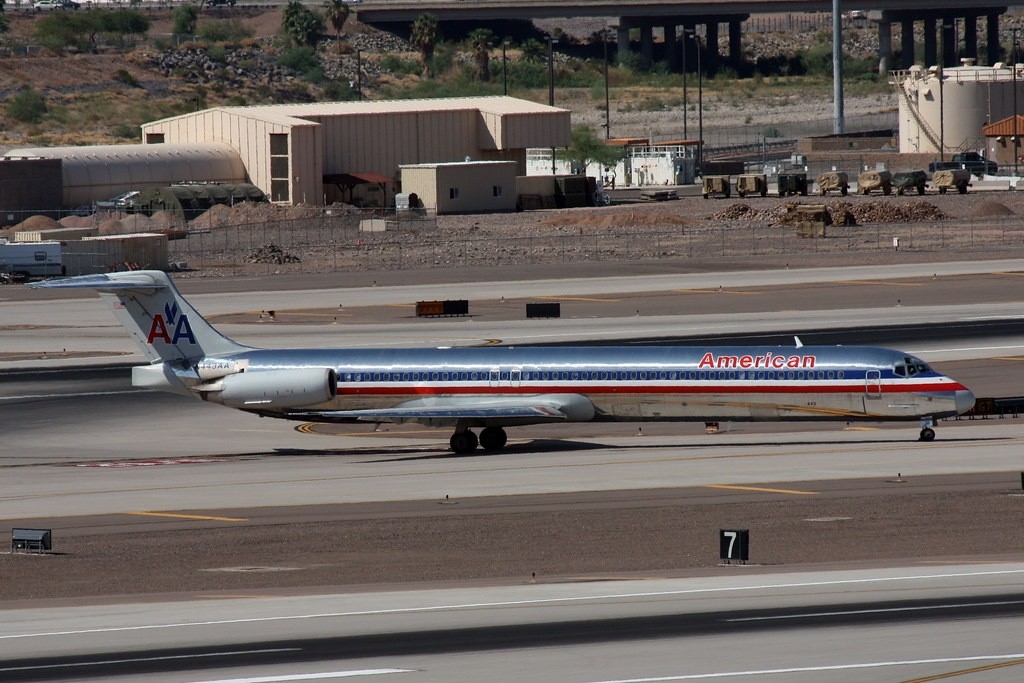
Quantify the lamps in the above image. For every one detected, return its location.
[894,237,899,247]
[11,527,52,555]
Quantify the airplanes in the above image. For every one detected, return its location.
[23,270,978,451]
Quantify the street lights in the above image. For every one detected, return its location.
[1009,27,1021,172]
[549,40,560,106]
[603,32,618,139]
[691,34,703,168]
[502,40,510,95]
[683,28,695,140]
[358,49,365,100]
[940,24,954,161]
[543,35,554,106]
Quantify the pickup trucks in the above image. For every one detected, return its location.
[930,151,997,178]
